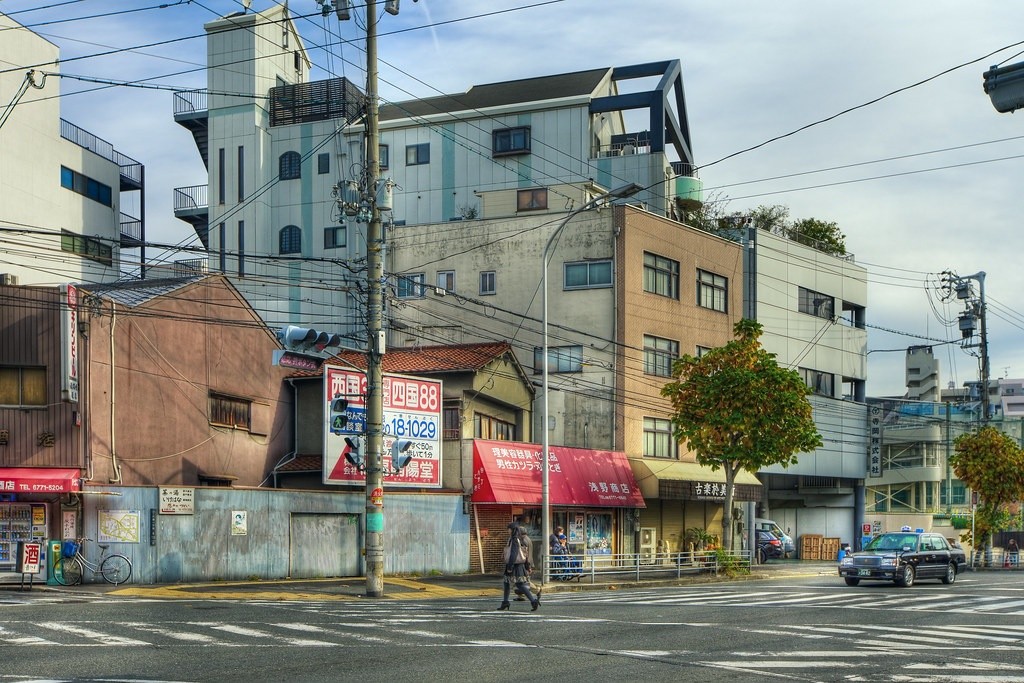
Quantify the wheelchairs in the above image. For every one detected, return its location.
[551,556,583,581]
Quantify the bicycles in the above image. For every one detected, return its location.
[53,536,132,587]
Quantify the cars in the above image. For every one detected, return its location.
[839,525,967,587]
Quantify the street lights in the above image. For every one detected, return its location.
[541,182,646,586]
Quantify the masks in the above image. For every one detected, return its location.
[558,533,563,536]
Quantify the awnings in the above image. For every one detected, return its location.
[471,439,647,508]
[626,456,763,502]
[0,468,80,492]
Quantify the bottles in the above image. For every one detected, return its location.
[0,508,31,561]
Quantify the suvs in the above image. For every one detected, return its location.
[743,529,785,564]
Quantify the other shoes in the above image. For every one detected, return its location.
[537,587,542,599]
[513,596,526,601]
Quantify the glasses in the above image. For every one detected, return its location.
[563,539,565,540]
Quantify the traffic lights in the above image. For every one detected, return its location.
[345,433,359,466]
[392,438,412,468]
[281,324,344,358]
[331,396,348,430]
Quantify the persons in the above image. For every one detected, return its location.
[836,546,850,562]
[496,523,542,612]
[784,543,791,558]
[592,516,599,531]
[1007,539,1019,565]
[549,526,582,575]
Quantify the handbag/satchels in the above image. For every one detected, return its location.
[501,538,526,564]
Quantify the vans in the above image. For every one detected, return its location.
[737,517,794,554]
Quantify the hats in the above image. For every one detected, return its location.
[560,535,567,539]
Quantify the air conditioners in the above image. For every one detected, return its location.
[0,274,19,285]
[634,527,656,565]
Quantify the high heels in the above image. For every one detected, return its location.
[497,601,510,610]
[531,599,541,611]
